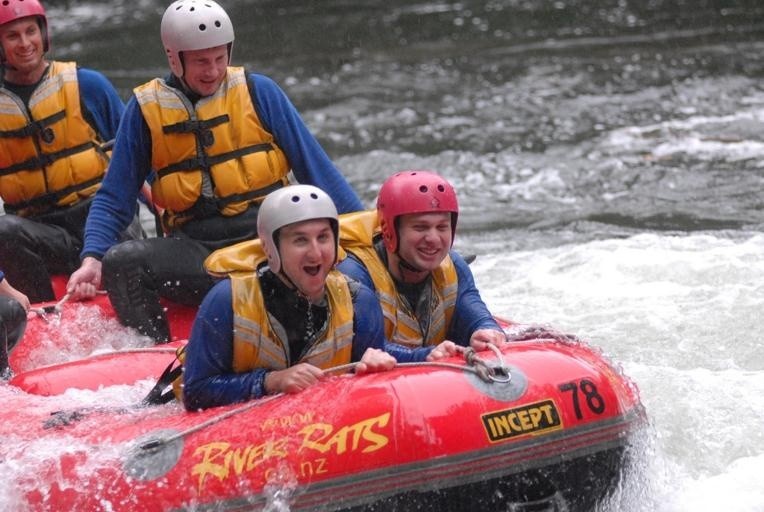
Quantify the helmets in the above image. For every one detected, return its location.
[377,169,458,254]
[256,183,339,275]
[161,0,234,78]
[0,0,50,52]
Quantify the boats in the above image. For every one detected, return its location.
[0,272,656,511]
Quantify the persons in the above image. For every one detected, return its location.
[165,183,398,414]
[1,0,155,307]
[335,169,507,364]
[64,1,369,347]
[0,271,31,375]
